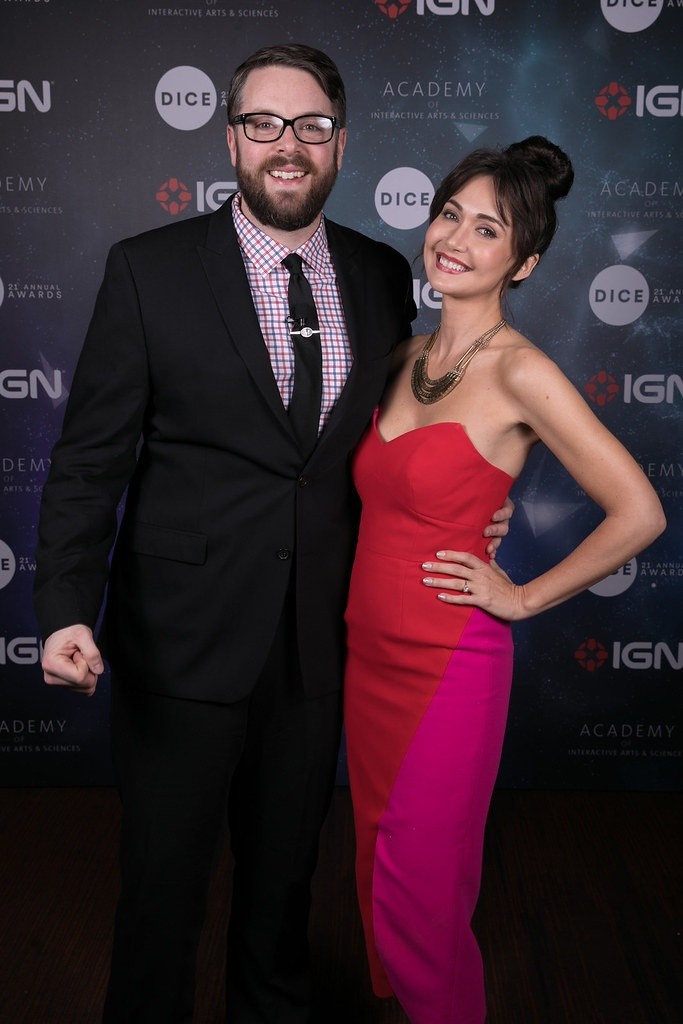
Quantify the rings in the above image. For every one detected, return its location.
[463,580,469,592]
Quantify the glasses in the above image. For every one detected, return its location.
[230,113,340,145]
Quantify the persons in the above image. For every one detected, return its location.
[32,43,419,1024]
[342,136,668,1024]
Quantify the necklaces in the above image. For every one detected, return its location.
[411,320,508,404]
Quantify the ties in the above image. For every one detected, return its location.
[281,254,322,454]
